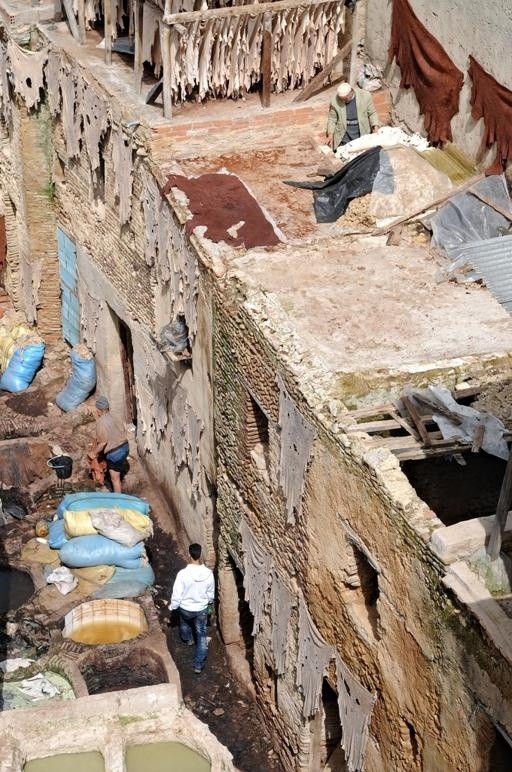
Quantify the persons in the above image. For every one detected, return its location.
[87,395,129,493]
[325,82,378,151]
[167,543,215,673]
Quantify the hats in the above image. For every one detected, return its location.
[337,83,351,96]
[95,396,109,409]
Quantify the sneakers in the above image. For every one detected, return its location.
[194,667,201,673]
[187,640,194,646]
